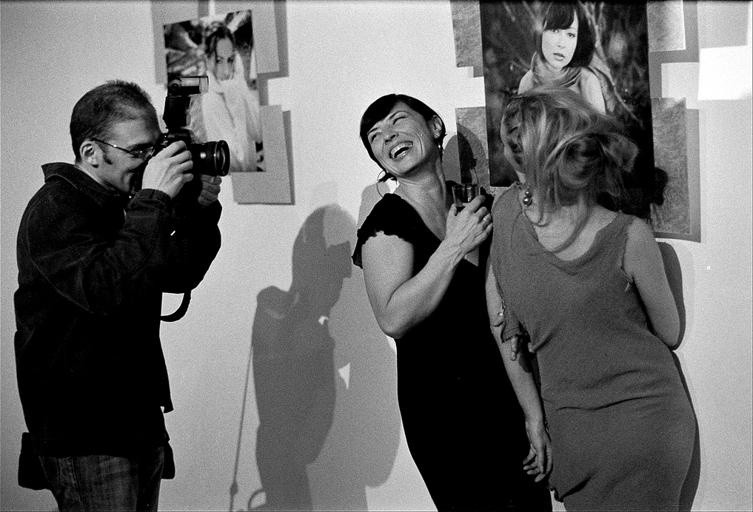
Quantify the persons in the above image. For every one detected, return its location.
[485,87,696,512]
[168,22,262,171]
[351,94,553,512]
[13,82,221,512]
[517,1,606,116]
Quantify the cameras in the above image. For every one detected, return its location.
[161,69,231,195]
[443,178,494,219]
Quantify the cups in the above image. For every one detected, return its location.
[450,185,482,214]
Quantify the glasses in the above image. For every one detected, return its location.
[88,137,155,161]
